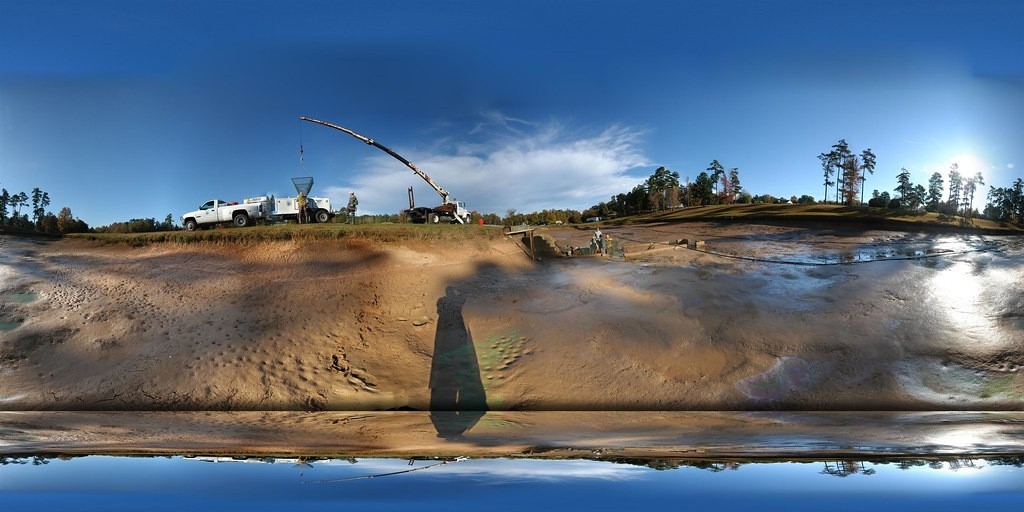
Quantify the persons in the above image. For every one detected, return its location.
[296,192,309,224]
[345,191,358,225]
[590,227,613,255]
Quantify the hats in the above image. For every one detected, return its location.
[299,192,304,196]
[349,191,354,195]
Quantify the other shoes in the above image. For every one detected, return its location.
[344,222,350,224]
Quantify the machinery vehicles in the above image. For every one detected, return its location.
[299,115,471,224]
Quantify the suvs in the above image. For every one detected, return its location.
[535,220,548,226]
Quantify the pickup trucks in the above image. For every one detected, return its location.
[180,199,263,232]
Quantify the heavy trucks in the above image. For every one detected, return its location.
[242,193,332,225]
